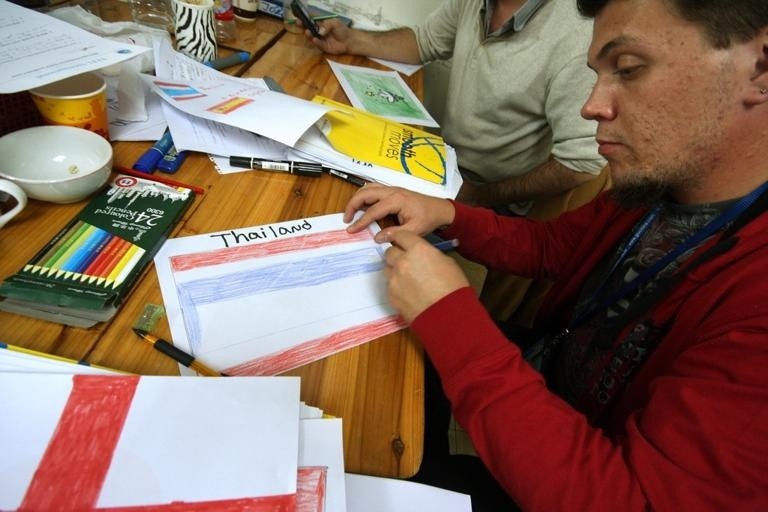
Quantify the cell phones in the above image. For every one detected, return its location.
[290,0,321,40]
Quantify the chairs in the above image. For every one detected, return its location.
[474,161,618,328]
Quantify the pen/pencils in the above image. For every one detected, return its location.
[284,14,339,24]
[22,217,149,291]
[323,168,368,187]
[133,327,226,376]
[433,239,460,251]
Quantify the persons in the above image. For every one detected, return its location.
[297,0,608,213]
[342,0,767,512]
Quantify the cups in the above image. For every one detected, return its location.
[26,72,110,142]
[232,1,258,23]
[174,1,217,63]
[128,1,174,35]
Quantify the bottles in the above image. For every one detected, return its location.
[214,0,239,44]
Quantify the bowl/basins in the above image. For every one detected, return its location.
[0,125,112,205]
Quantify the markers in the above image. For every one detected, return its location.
[132,131,174,174]
[230,157,321,176]
[203,52,250,70]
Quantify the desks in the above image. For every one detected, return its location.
[2,0,427,483]
